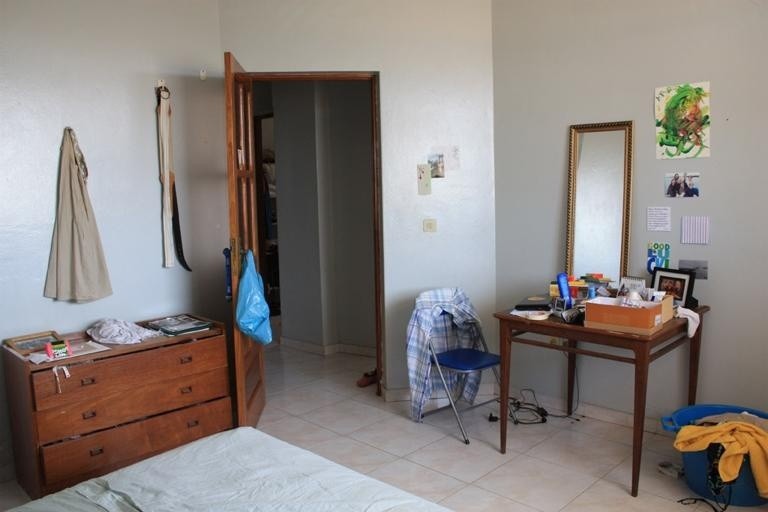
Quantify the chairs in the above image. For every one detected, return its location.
[415,287,518,446]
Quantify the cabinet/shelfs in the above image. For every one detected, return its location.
[2,313,236,499]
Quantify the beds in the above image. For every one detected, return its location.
[0,426,454,512]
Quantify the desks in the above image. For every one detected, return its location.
[492,304,711,497]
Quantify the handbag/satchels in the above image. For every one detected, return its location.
[235,248,272,346]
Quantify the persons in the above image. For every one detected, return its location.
[667,173,699,197]
[660,277,683,300]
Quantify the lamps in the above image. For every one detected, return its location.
[428,151,446,180]
[650,266,696,308]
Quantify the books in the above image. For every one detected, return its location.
[144,314,212,338]
[515,294,552,311]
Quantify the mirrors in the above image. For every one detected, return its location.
[566,120,633,278]
